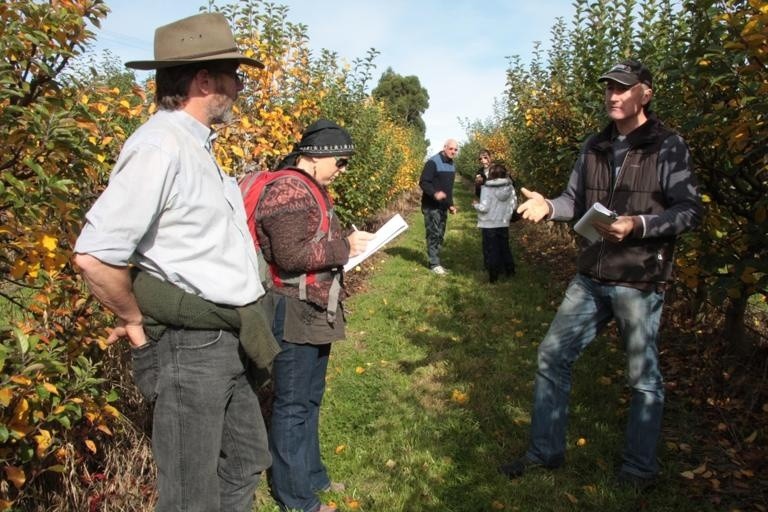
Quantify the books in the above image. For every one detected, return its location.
[341,212,409,274]
[573,201,615,244]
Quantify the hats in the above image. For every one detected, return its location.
[125,12,265,70]
[596,59,654,87]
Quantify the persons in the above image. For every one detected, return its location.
[475,149,492,205]
[255,121,351,510]
[69,15,270,510]
[468,161,519,284]
[503,60,702,490]
[416,136,461,278]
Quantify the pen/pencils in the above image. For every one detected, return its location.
[351,223,358,232]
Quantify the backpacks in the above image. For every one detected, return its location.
[236,169,330,286]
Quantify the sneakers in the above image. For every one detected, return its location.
[499,454,534,479]
[613,472,655,494]
[329,482,348,493]
[433,266,444,274]
[318,505,339,512]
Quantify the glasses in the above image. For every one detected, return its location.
[335,156,348,168]
